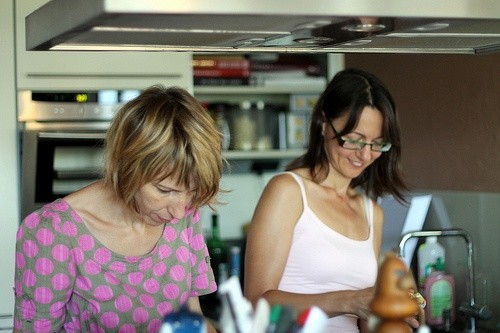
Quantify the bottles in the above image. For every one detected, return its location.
[206,212,227,281]
[418,236,446,282]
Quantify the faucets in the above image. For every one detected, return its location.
[397,228,480,333]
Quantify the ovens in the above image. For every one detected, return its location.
[14,50,195,224]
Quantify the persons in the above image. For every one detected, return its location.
[13,84,234,333]
[243,68,419,333]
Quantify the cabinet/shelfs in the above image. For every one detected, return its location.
[193,54,346,159]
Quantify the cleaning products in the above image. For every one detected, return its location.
[422,255,457,327]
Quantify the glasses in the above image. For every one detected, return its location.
[328,119,393,152]
